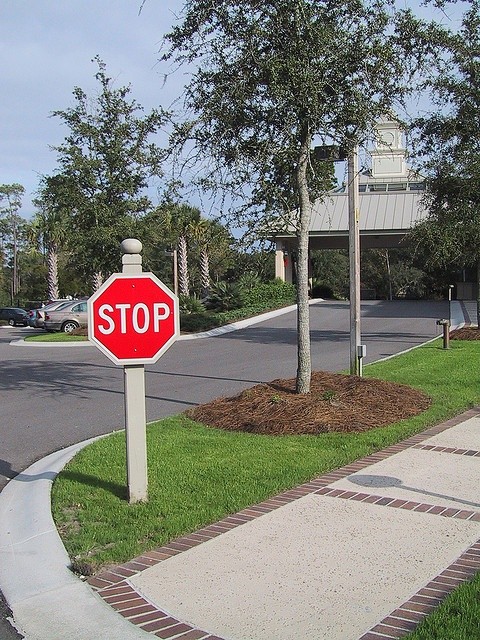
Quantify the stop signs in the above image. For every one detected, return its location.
[87,272,180,366]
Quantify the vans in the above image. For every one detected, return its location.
[0,307,27,326]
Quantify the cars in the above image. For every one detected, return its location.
[29,310,38,328]
[43,300,87,333]
[34,301,74,332]
[23,312,30,326]
